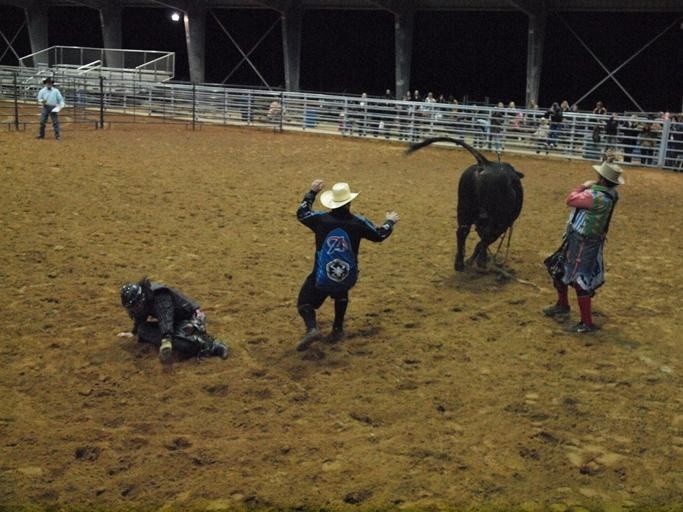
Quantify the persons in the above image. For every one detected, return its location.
[339,91,524,150]
[526,100,683,169]
[118,280,229,361]
[295,179,399,351]
[269,95,286,121]
[306,105,319,128]
[545,161,624,334]
[36,78,64,140]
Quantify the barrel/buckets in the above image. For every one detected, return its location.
[305,110,317,127]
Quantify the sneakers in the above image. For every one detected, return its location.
[214,339,229,361]
[543,304,572,316]
[320,328,345,345]
[564,322,597,333]
[295,328,319,352]
[34,134,62,142]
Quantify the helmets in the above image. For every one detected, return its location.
[120,283,148,315]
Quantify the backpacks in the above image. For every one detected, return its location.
[313,213,363,293]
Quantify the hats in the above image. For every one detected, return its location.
[42,77,55,85]
[319,181,362,210]
[592,159,626,184]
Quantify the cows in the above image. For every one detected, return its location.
[405,137,524,272]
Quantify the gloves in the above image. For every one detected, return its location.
[158,338,173,363]
